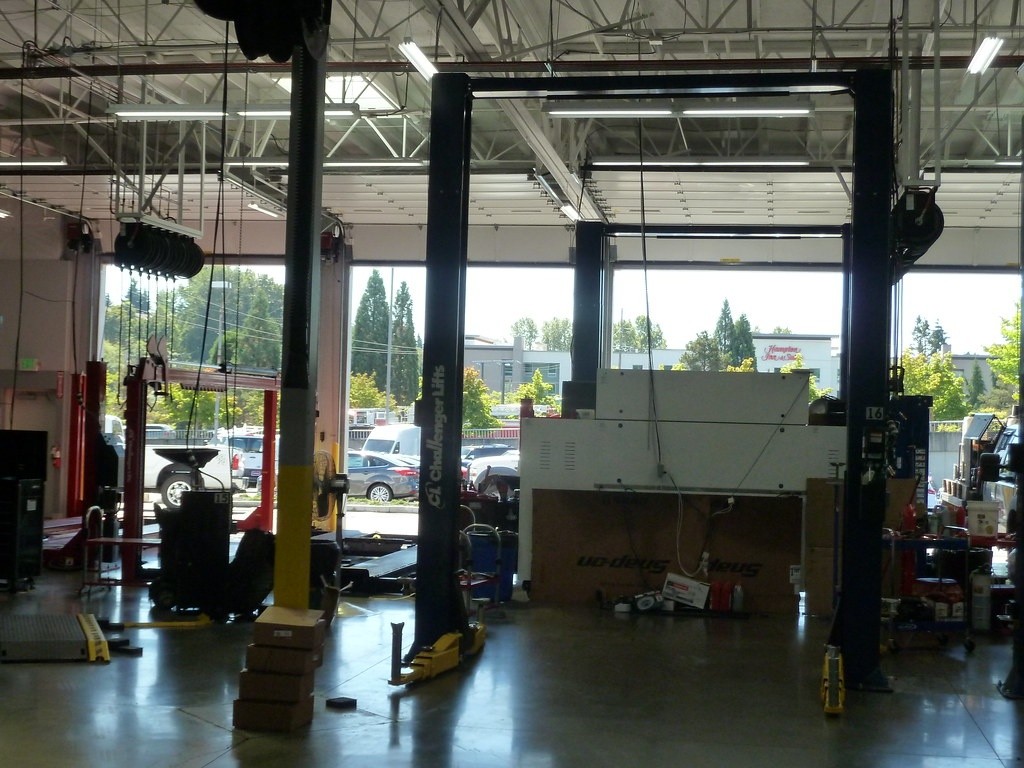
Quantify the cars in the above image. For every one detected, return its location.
[144,423,175,444]
[204,421,521,505]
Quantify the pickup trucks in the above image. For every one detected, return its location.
[104,413,250,510]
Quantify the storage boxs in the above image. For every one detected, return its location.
[233,698,314,733]
[245,642,324,674]
[805,477,916,548]
[253,606,325,648]
[662,572,711,610]
[809,412,847,426]
[805,548,894,618]
[239,667,314,702]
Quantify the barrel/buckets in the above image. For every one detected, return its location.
[967,500,1000,540]
[310,538,342,625]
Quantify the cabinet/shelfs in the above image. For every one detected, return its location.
[78,505,161,597]
[901,504,1016,635]
[881,525,977,653]
[457,524,501,615]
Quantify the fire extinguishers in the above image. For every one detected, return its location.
[970,563,992,630]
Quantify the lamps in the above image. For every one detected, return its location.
[236,103,361,121]
[966,37,1004,76]
[398,41,439,82]
[104,103,229,120]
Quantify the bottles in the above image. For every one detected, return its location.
[708,574,744,612]
[928,505,966,536]
[904,504,916,531]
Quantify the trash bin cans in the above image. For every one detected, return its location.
[468,531,518,604]
